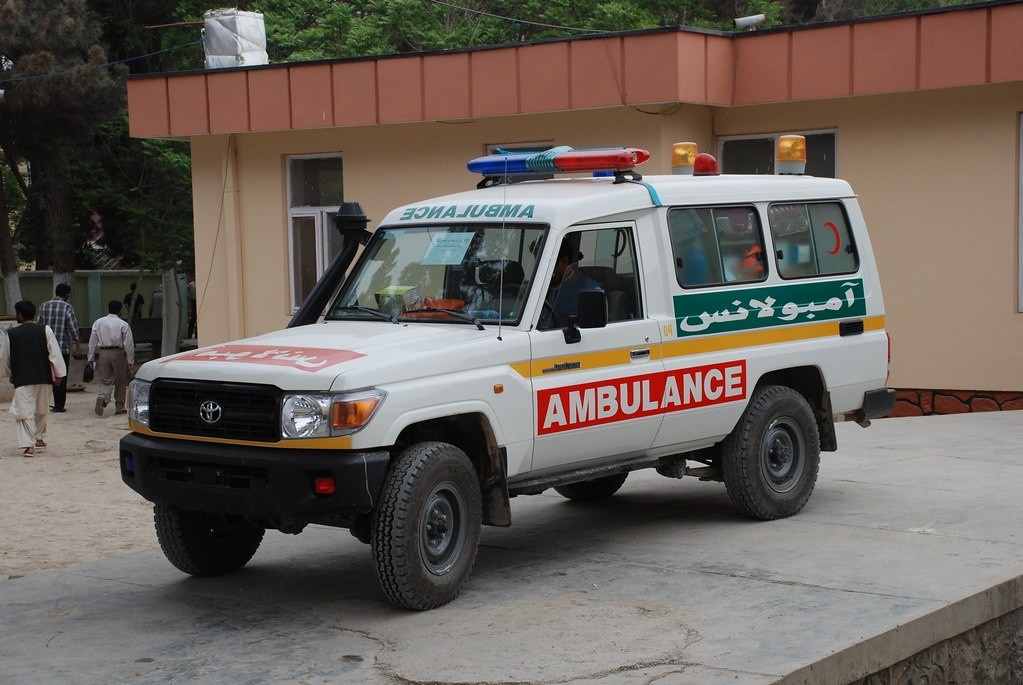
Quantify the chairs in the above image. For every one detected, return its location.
[461,260,525,312]
[580,266,627,320]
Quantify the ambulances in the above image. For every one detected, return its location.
[120,135,896,612]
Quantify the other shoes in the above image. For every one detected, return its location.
[53,407,66,412]
[95,397,104,415]
[115,409,127,415]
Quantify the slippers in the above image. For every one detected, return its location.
[23,448,35,456]
[35,440,47,449]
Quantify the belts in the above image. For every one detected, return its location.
[100,346,122,350]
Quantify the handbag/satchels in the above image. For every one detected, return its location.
[83,362,94,382]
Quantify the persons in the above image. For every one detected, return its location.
[87,300,134,415]
[150,281,196,352]
[0,300,66,457]
[470,235,606,322]
[123,283,145,320]
[37,283,82,413]
